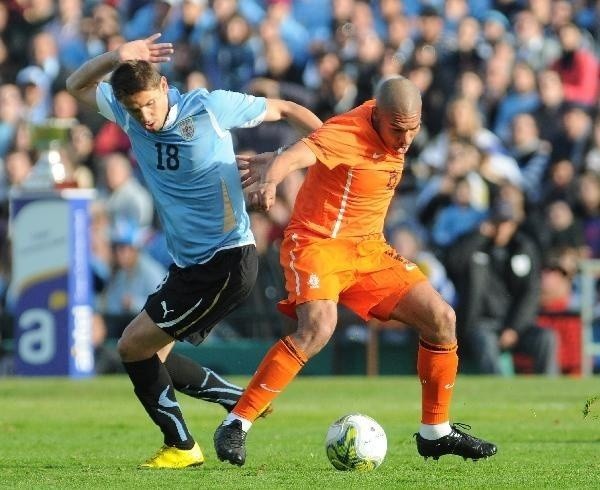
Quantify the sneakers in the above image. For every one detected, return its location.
[416,425,497,462]
[213,419,247,467]
[137,441,204,470]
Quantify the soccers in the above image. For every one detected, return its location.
[326,414,387,471]
